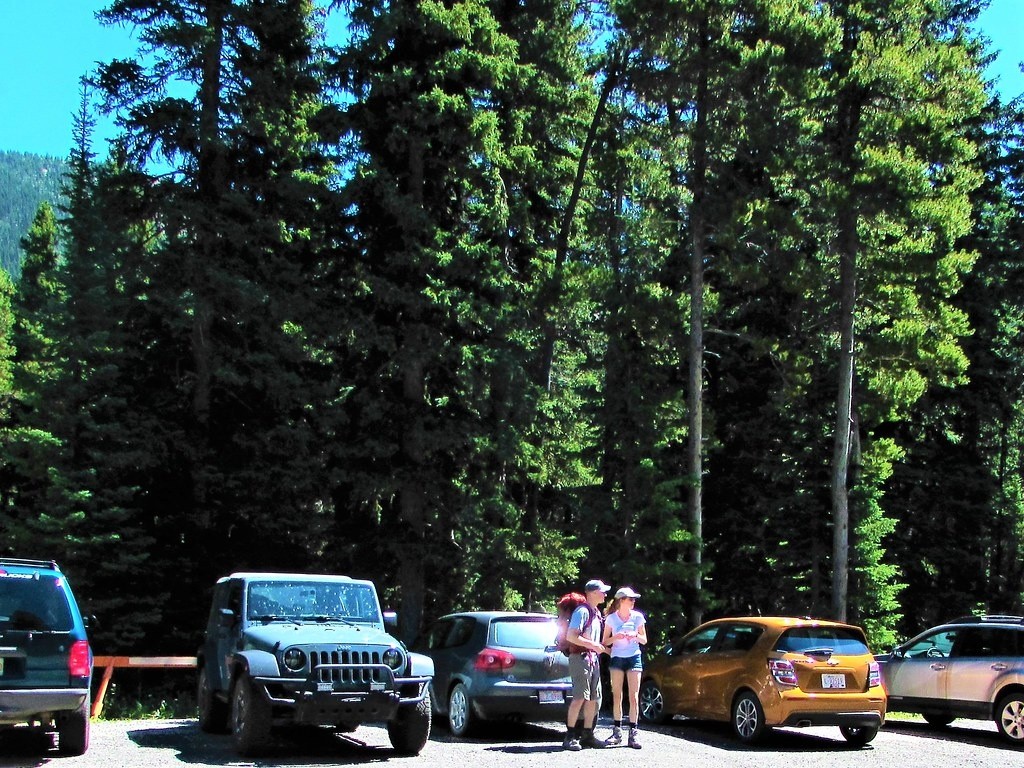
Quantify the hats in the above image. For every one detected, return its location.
[614,587,641,602]
[584,580,611,592]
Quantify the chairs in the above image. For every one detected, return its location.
[730,631,757,651]
[9,597,57,631]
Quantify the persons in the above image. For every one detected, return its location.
[602,587,648,749]
[561,579,612,751]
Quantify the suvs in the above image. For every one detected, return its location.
[197,571,435,759]
[0,556,97,756]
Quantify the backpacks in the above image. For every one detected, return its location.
[557,593,602,656]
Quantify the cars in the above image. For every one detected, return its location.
[880,614,1024,747]
[406,610,600,740]
[638,616,887,747]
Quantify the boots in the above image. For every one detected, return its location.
[628,728,642,749]
[605,726,623,745]
[579,729,605,749]
[562,732,582,751]
